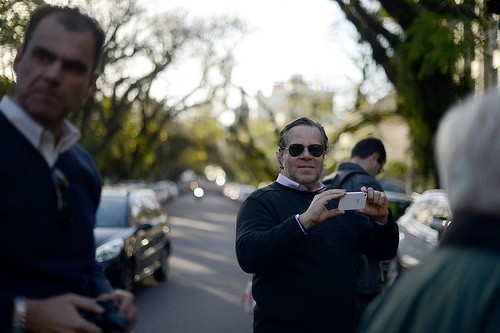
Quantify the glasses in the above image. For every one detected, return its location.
[283,144,326,157]
[379,163,384,173]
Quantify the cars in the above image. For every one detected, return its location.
[93,186,171,292]
[389,187,452,269]
[376,179,414,220]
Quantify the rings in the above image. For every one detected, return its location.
[380,194,386,199]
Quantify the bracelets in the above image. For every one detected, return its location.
[295,213,308,233]
[13,297,26,332]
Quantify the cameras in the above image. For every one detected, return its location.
[77,299,131,333]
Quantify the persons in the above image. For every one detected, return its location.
[357,86,499,333]
[323,138,386,301]
[0,5,136,333]
[235,116,400,333]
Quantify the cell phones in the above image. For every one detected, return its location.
[337,192,367,211]
